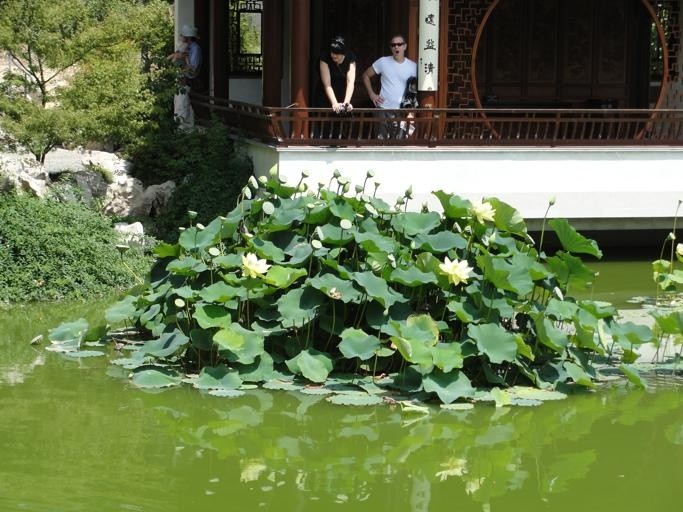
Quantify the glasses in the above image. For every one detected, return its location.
[389,43,405,47]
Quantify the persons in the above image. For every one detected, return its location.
[312,35,358,146]
[359,31,418,142]
[166,25,207,94]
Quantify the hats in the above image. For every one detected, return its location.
[328,36,347,54]
